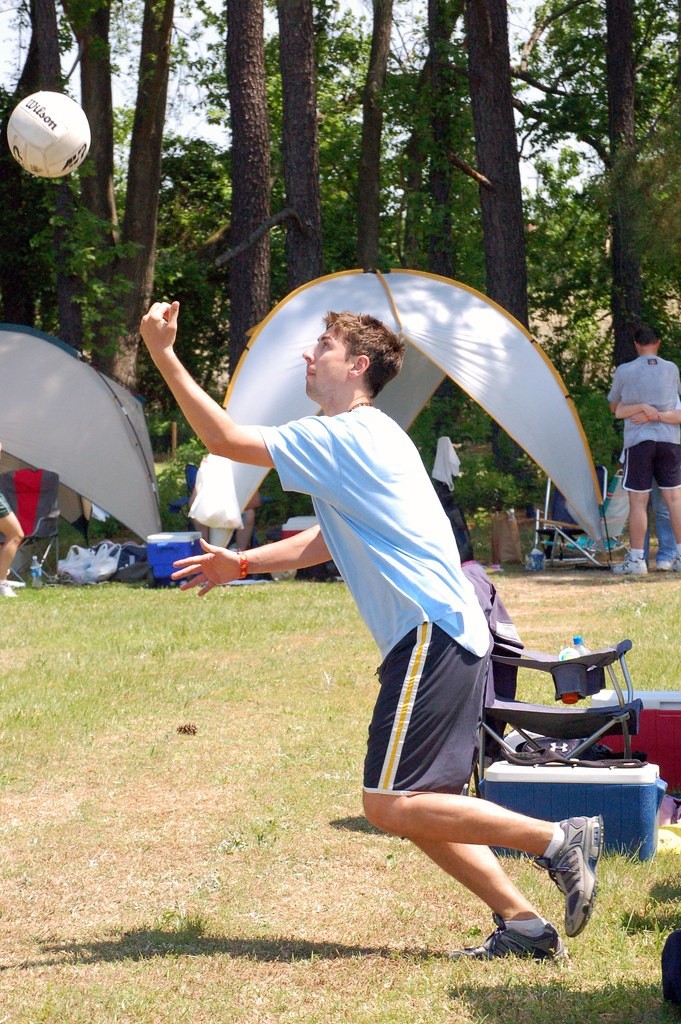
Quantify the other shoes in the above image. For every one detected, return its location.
[655,559,674,570]
[0,581,24,597]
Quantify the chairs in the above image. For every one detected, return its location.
[0,467,61,585]
[166,464,280,549]
[534,465,613,571]
[462,560,649,798]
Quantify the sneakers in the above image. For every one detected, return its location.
[531,815,603,937]
[674,551,681,571]
[613,548,648,574]
[449,913,564,961]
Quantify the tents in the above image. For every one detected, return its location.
[187,267,612,575]
[0,320,163,543]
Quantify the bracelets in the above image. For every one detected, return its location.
[238,549,248,580]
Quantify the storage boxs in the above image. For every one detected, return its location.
[590,689,681,792]
[479,761,668,863]
[281,516,319,541]
[146,531,204,578]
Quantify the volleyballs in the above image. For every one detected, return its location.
[8,91,92,177]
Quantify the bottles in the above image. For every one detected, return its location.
[572,636,591,656]
[29,556,43,588]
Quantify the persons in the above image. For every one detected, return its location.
[138,300,603,962]
[607,326,681,576]
[0,493,25,598]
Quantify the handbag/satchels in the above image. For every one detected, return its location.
[513,735,648,762]
[58,542,122,585]
